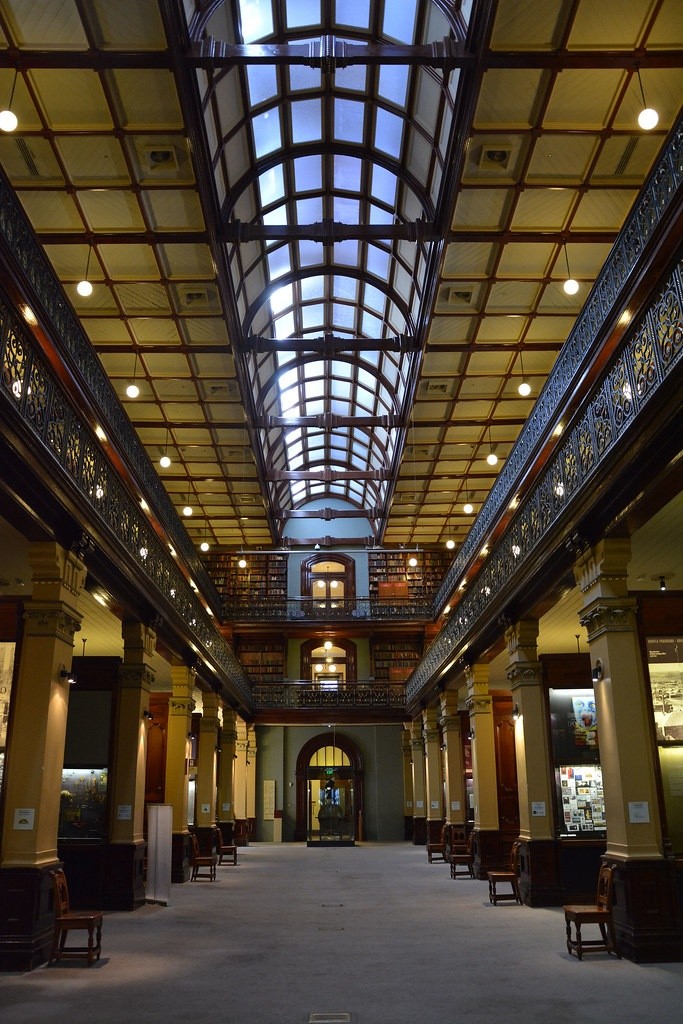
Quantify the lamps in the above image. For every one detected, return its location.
[315,641,338,672]
[408,704,519,768]
[239,543,246,567]
[633,71,660,129]
[446,514,455,548]
[659,575,666,591]
[562,236,580,295]
[160,421,172,467]
[409,535,417,566]
[484,421,498,465]
[0,68,20,133]
[125,344,141,398]
[57,670,251,765]
[463,473,472,513]
[183,479,193,516]
[199,516,210,551]
[592,665,601,683]
[517,343,531,397]
[77,239,95,296]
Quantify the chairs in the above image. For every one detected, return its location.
[189,835,217,883]
[217,826,238,866]
[451,832,478,879]
[488,842,524,906]
[562,862,620,961]
[47,868,103,969]
[318,804,344,840]
[426,825,447,863]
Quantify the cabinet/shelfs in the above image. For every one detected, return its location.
[202,555,287,619]
[232,637,289,703]
[368,553,454,616]
[368,633,426,690]
